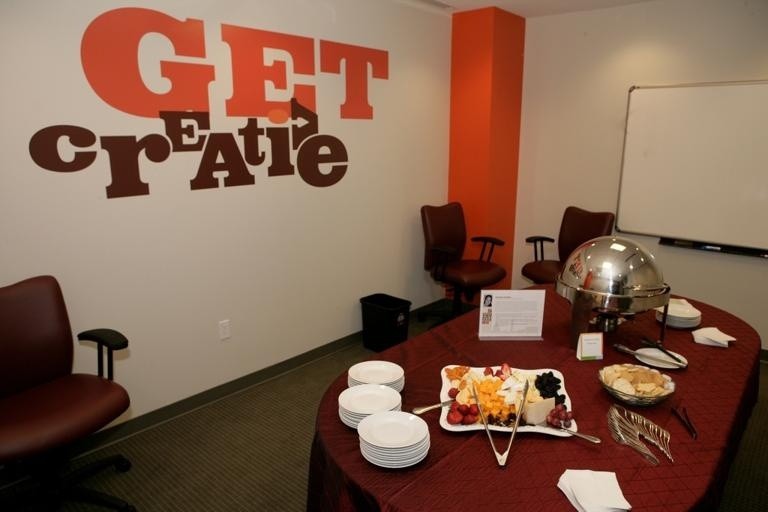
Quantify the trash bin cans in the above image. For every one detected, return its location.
[360,293,412,353]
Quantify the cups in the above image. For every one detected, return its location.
[595,314,618,332]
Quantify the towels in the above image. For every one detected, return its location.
[690,323,738,350]
[556,461,634,512]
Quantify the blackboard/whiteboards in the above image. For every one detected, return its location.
[614,79,768,259]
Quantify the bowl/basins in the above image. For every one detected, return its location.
[597,363,675,408]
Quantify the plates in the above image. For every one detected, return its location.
[337,360,406,430]
[655,306,703,328]
[438,364,578,438]
[357,410,432,469]
[632,346,688,370]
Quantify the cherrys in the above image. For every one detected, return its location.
[448,388,478,424]
[546,404,573,428]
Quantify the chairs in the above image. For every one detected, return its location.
[420,201,507,332]
[521,205,616,284]
[0,274,141,512]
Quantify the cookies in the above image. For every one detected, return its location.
[605,367,665,399]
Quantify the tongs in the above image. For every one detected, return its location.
[471,377,529,466]
[613,336,688,369]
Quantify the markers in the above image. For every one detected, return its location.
[703,245,721,251]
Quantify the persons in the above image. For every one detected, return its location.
[484,294,493,308]
